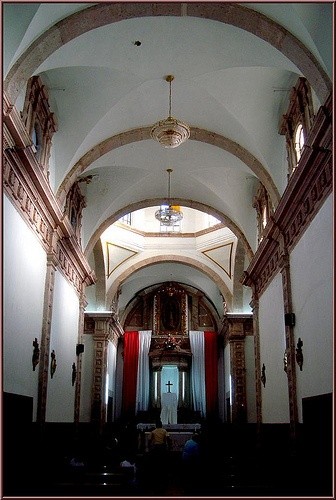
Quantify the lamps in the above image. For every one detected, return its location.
[51,350,57,377]
[261,364,266,387]
[72,363,78,385]
[32,337,40,371]
[151,75,190,148]
[295,337,303,371]
[155,169,182,226]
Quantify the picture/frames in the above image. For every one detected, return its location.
[153,292,188,338]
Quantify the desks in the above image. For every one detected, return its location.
[137,423,201,450]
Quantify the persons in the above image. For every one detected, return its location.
[181,432,202,469]
[144,420,176,456]
